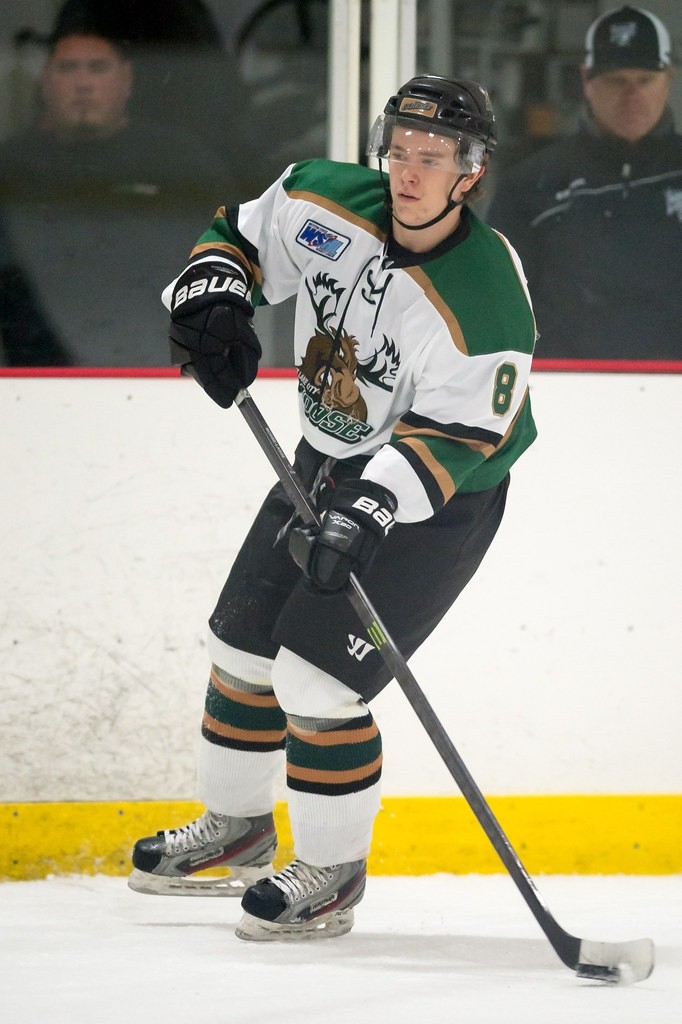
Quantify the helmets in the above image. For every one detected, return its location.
[384,74,498,151]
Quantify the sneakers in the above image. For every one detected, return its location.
[128,809,277,897]
[235,857,366,941]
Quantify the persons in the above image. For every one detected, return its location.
[131,73,538,942]
[484,1,681,360]
[0,13,251,367]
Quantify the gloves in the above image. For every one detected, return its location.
[288,478,398,599]
[168,260,263,409]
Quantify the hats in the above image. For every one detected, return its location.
[584,4,672,71]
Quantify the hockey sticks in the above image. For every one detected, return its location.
[228,386,659,989]
